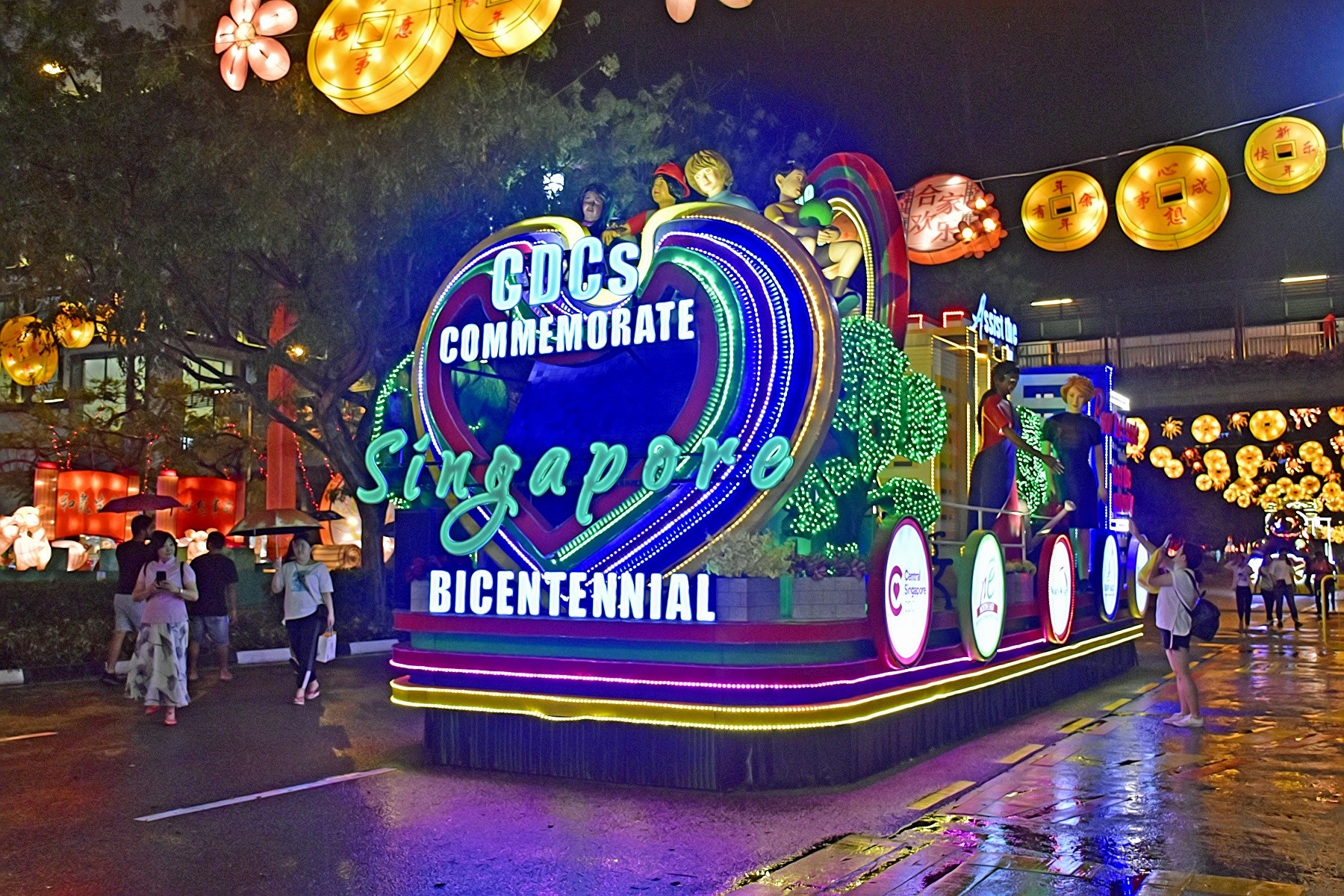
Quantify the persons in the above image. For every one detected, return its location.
[602,161,689,245]
[186,532,238,679]
[1128,519,1203,727]
[272,535,334,704]
[1254,554,1276,625]
[1300,548,1315,596]
[1310,551,1338,614]
[575,181,614,235]
[1269,549,1304,629]
[123,531,198,724]
[764,162,862,317]
[685,150,760,213]
[1224,555,1254,633]
[966,362,1064,570]
[102,515,155,684]
[1040,376,1107,591]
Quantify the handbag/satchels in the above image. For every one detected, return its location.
[317,627,337,662]
[317,603,328,618]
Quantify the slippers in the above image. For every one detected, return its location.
[306,690,323,698]
[294,696,304,705]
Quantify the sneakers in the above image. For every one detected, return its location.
[1172,715,1203,727]
[1163,713,1184,724]
[101,670,121,685]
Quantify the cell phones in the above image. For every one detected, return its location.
[156,571,166,587]
[1163,534,1172,549]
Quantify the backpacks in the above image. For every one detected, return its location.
[1174,570,1220,641]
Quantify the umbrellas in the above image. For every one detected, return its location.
[98,494,184,516]
[226,508,325,562]
[305,510,345,522]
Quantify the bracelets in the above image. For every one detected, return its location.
[178,587,183,595]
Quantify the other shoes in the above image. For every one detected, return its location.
[165,712,176,725]
[1294,621,1303,630]
[145,706,160,715]
[289,658,301,674]
[1277,621,1283,628]
